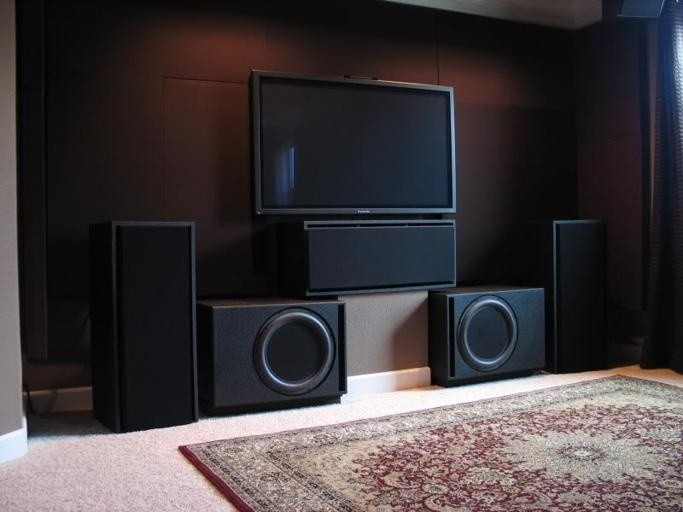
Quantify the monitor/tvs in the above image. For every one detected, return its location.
[246,68,458,215]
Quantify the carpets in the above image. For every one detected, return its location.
[175,373,683,512]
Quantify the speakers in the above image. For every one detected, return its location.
[197,297,348,417]
[88,218,199,432]
[552,219,612,373]
[428,283,546,388]
